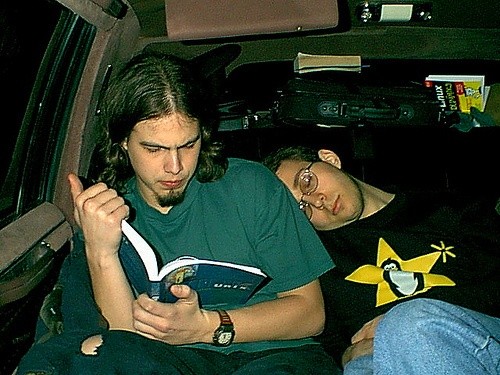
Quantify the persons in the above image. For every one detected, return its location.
[262,145,500,375]
[16,53,342,374]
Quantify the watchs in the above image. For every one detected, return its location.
[212,309,235,348]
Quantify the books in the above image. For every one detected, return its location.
[294,52,361,74]
[118,219,267,310]
[424,75,490,113]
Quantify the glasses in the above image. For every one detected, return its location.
[297,161,319,221]
